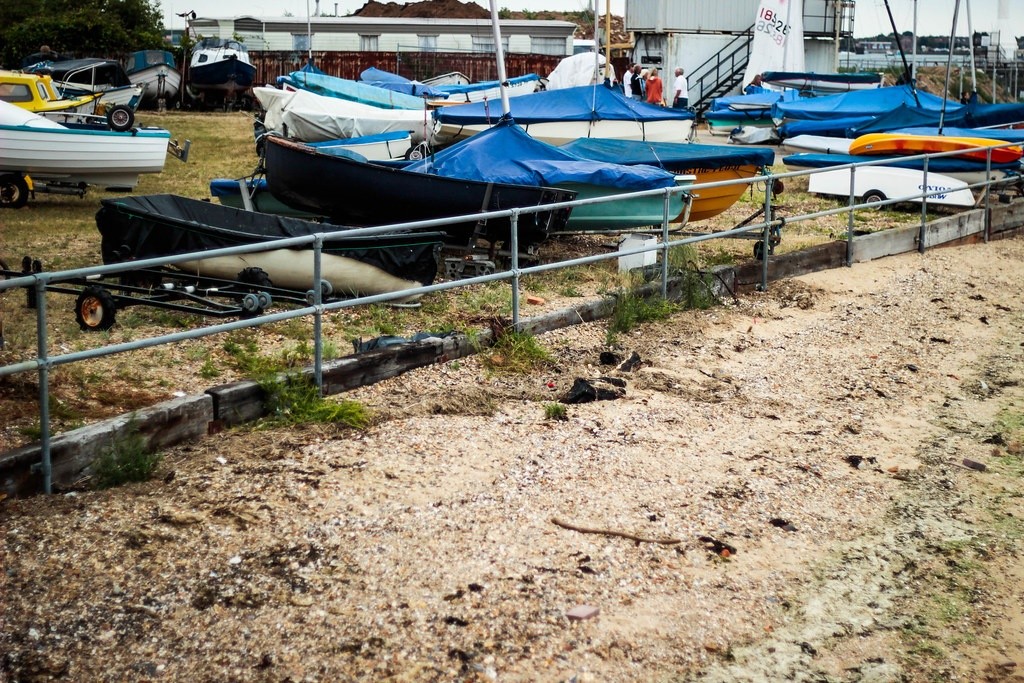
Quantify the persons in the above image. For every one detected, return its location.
[960,90,978,105]
[623,63,664,108]
[672,68,688,109]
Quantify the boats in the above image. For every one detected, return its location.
[95,192,454,305]
[0,0,1024,238]
